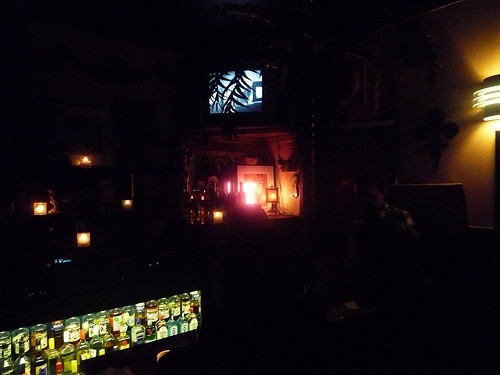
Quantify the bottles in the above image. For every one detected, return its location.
[59,331,78,375]
[0,331,12,360]
[89,328,103,357]
[81,313,98,341]
[48,320,64,351]
[102,322,116,355]
[116,291,201,351]
[14,341,32,375]
[108,307,122,337]
[30,323,49,357]
[0,343,15,375]
[75,329,92,375]
[44,338,62,375]
[12,327,30,361]
[32,338,48,375]
[65,316,81,345]
[96,310,110,339]
[182,180,245,225]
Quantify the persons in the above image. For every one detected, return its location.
[105,163,484,374]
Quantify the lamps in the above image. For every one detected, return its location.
[472,74,500,121]
[266,185,280,213]
